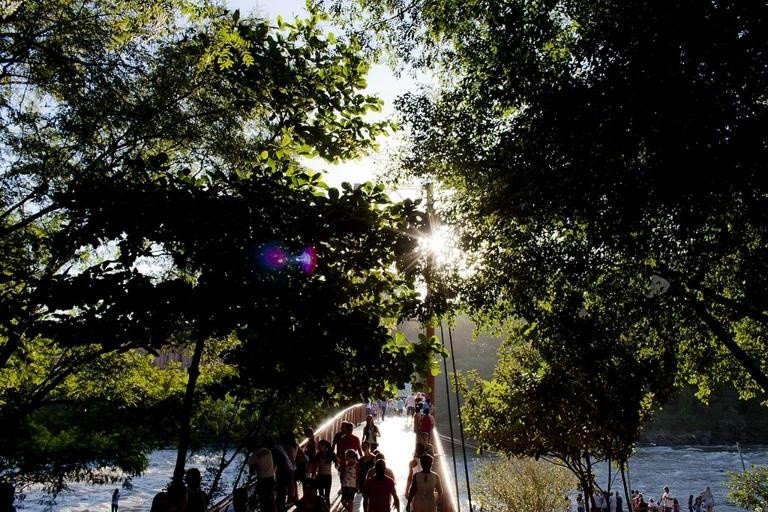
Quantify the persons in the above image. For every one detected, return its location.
[560,483,716,511]
[109,487,123,512]
[1,481,18,512]
[182,388,445,512]
